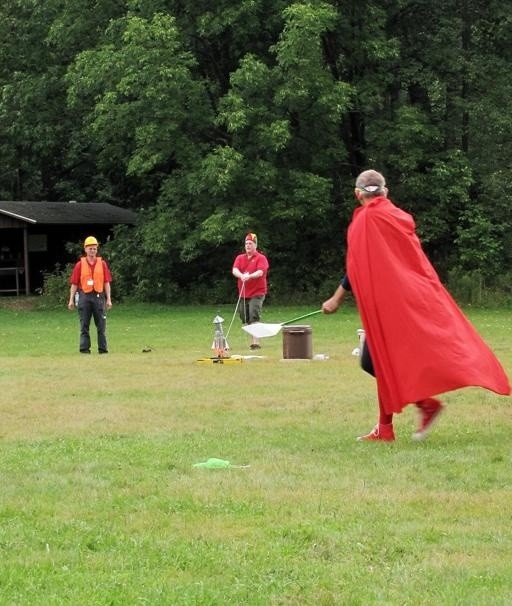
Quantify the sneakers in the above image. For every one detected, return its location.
[411,398,443,438]
[80,349,90,353]
[99,348,107,353]
[356,423,395,440]
[250,344,260,349]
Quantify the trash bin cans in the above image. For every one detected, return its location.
[282,325,313,360]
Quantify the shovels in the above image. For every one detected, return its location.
[241,310,323,338]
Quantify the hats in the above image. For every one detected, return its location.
[355,170,386,192]
[246,233,257,242]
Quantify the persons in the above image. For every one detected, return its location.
[66,235,114,354]
[231,232,270,351]
[320,166,512,444]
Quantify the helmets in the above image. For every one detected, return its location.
[84,236,98,247]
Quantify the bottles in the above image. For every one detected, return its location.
[74,291,80,307]
